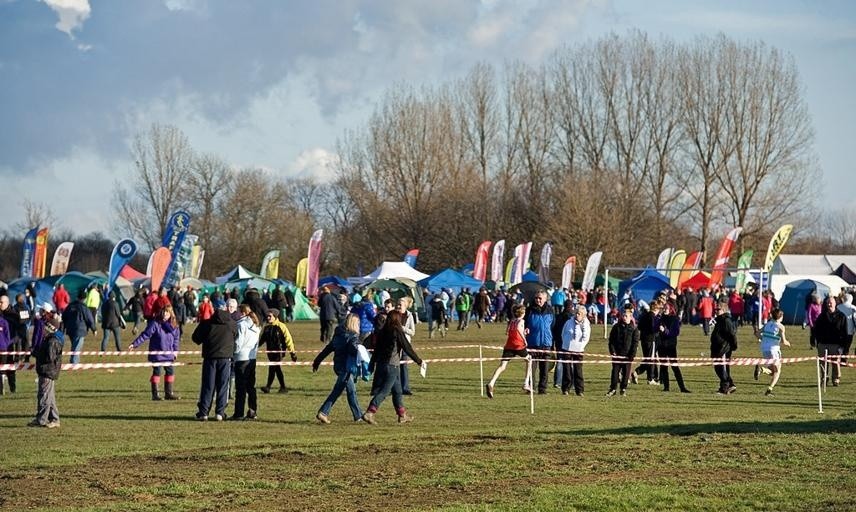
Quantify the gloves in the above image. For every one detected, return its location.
[290,352,297,361]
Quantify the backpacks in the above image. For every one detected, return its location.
[457,295,464,305]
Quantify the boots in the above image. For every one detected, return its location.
[165,375,180,400]
[151,376,162,400]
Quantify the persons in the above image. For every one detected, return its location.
[84,291,96,326]
[123,289,145,335]
[803,288,817,329]
[837,294,856,366]
[525,288,556,395]
[242,283,295,308]
[552,299,575,388]
[225,298,244,399]
[172,305,191,335]
[70,283,97,291]
[27,318,63,428]
[52,283,70,333]
[671,303,691,324]
[486,286,524,304]
[396,296,415,395]
[654,303,691,393]
[97,282,108,309]
[839,285,856,294]
[319,313,346,342]
[754,286,780,308]
[587,306,601,325]
[317,286,396,313]
[486,304,514,323]
[257,308,297,393]
[711,302,738,395]
[668,286,754,303]
[251,308,261,324]
[261,308,294,324]
[605,309,639,397]
[691,303,710,336]
[561,306,591,395]
[27,280,52,318]
[423,286,486,338]
[552,286,649,306]
[631,301,660,385]
[100,290,123,357]
[0,280,27,396]
[649,290,668,384]
[191,300,215,322]
[145,283,191,305]
[191,300,239,420]
[738,303,754,327]
[486,304,536,398]
[636,306,649,316]
[191,283,240,301]
[312,313,364,424]
[615,303,636,382]
[810,297,850,387]
[27,319,44,357]
[144,305,165,325]
[821,293,840,314]
[361,310,423,426]
[62,291,96,363]
[754,309,777,343]
[838,294,846,297]
[128,305,180,401]
[754,308,791,398]
[361,314,388,347]
[227,303,261,420]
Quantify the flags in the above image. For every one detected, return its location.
[654,224,794,295]
[404,249,420,272]
[472,241,602,289]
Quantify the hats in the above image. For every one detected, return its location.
[323,286,330,292]
[267,308,279,319]
[36,302,53,313]
[43,318,59,333]
[811,294,819,302]
[213,299,227,309]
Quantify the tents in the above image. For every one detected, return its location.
[832,293,839,296]
[767,253,855,325]
[108,283,145,290]
[780,290,802,325]
[817,290,821,304]
[803,290,811,321]
[38,264,295,283]
[295,286,319,320]
[821,290,839,292]
[318,261,484,321]
[821,293,827,304]
[618,265,669,308]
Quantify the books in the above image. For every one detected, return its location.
[420,360,426,379]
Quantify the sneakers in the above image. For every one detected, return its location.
[833,379,840,388]
[715,390,727,395]
[486,384,494,398]
[754,365,762,380]
[765,390,775,398]
[214,414,223,420]
[604,389,616,397]
[522,384,535,393]
[578,393,584,396]
[193,414,207,421]
[554,384,560,388]
[46,420,60,428]
[647,379,660,386]
[261,387,270,394]
[227,416,244,420]
[245,416,257,421]
[403,391,413,395]
[681,390,691,393]
[727,385,736,393]
[632,371,638,385]
[539,388,549,394]
[27,419,41,427]
[398,414,414,423]
[563,390,569,395]
[316,413,331,424]
[278,387,288,393]
[662,388,669,391]
[361,411,377,424]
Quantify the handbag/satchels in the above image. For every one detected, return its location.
[119,316,126,329]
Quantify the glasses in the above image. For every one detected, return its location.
[535,296,544,301]
